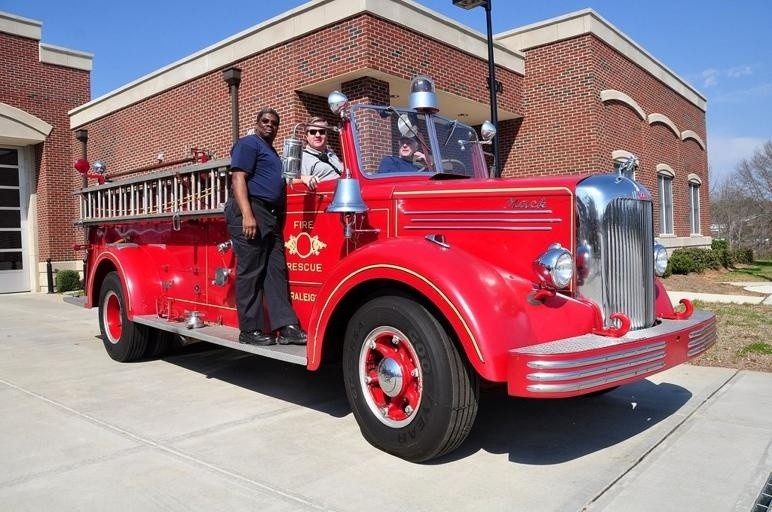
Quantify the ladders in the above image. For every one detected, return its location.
[72,158,232,225]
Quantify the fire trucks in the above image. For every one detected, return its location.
[71,74,715,462]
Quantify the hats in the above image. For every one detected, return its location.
[399,134,419,145]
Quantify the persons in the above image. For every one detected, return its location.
[300,116,343,191]
[224,108,308,347]
[378,132,433,175]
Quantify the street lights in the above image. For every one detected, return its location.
[453,0,500,179]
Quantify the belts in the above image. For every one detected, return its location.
[229,192,277,216]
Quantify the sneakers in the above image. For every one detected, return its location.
[277,324,308,345]
[239,330,276,345]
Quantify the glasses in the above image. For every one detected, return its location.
[259,118,279,125]
[308,128,327,135]
